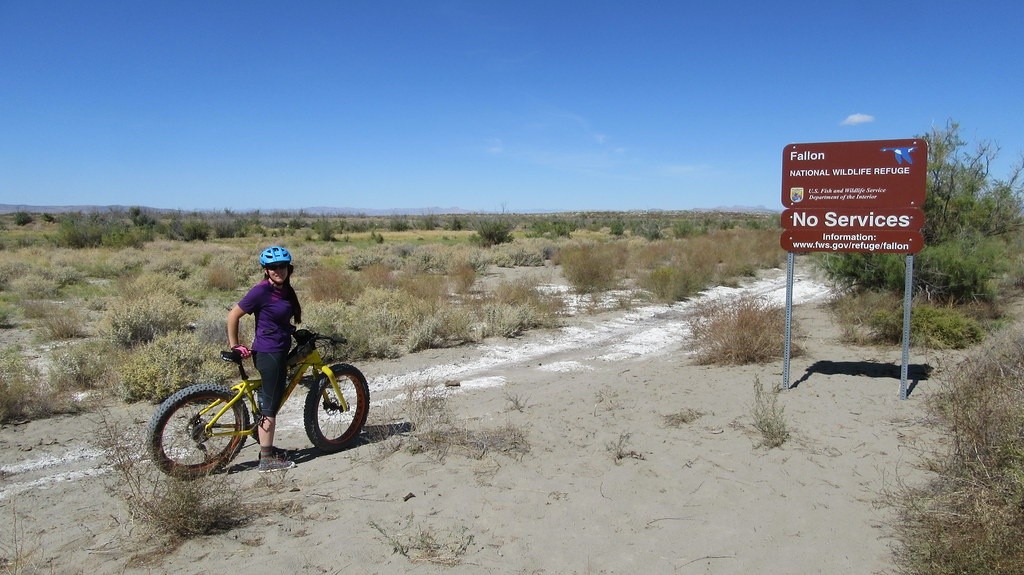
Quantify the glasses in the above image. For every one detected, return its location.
[265,263,288,270]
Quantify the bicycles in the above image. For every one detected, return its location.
[145,333,370,481]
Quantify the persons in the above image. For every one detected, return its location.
[227,246,303,473]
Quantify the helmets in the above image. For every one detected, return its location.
[258,246,292,267]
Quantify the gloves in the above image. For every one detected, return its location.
[290,325,296,335]
[230,345,250,359]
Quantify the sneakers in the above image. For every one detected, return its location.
[258,444,290,462]
[258,458,296,472]
